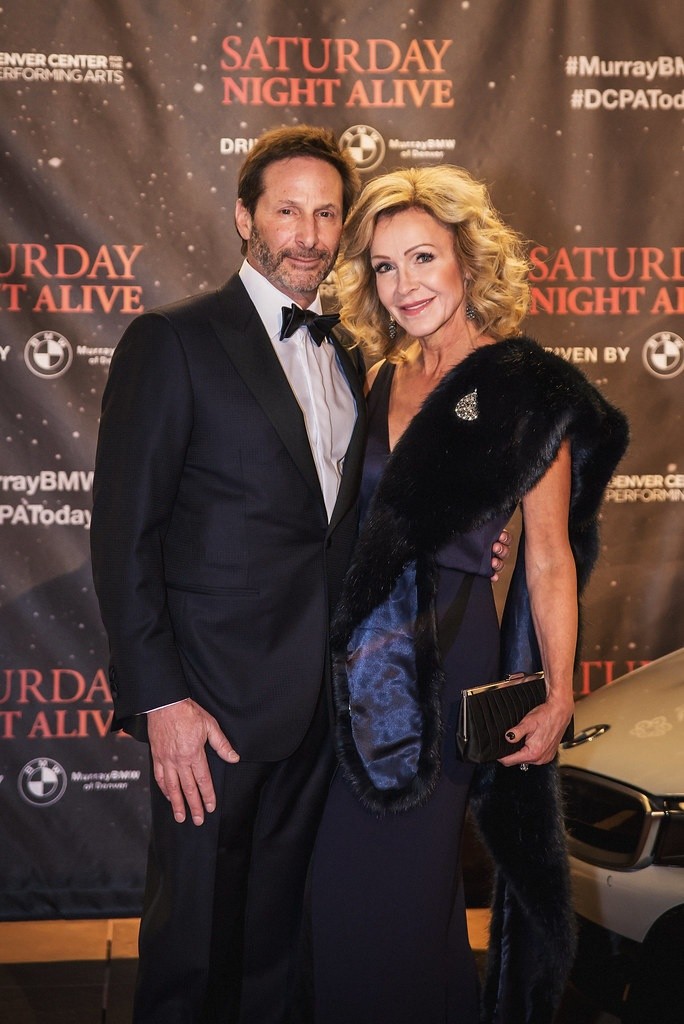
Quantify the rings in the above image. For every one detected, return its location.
[520,763,528,771]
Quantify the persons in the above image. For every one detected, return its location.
[306,166,629,1024]
[92,125,513,1024]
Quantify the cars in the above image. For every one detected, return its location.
[550,648,684,1024]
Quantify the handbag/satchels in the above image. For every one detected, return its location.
[456,671,576,765]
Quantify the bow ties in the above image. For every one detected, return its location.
[280,303,340,347]
